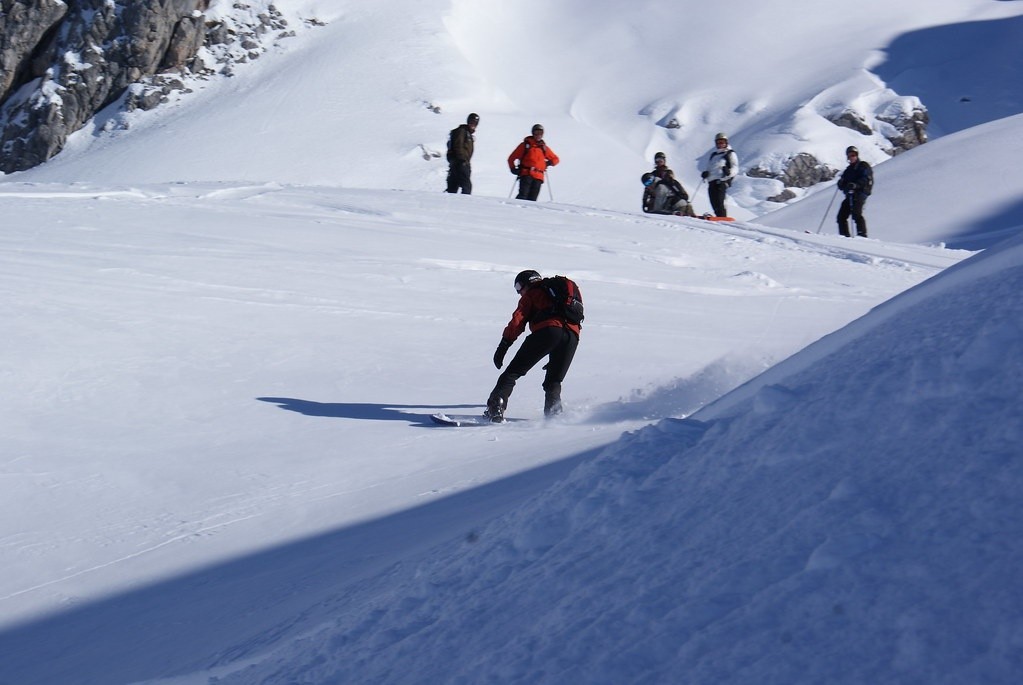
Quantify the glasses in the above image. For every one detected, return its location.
[534,130,543,135]
[848,154,857,158]
[514,283,522,292]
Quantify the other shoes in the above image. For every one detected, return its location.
[483,404,505,423]
[857,231,867,237]
[544,401,563,417]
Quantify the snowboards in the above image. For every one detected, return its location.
[430,415,584,426]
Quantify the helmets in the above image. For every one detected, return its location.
[514,270,541,293]
[532,124,544,134]
[467,113,479,126]
[847,146,859,156]
[641,173,655,186]
[655,152,666,159]
[715,133,728,148]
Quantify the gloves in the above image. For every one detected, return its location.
[494,337,513,369]
[701,170,708,178]
[511,168,520,174]
[839,179,846,188]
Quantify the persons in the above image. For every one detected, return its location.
[483,270,582,423]
[642,152,695,216]
[446,113,479,194]
[702,133,739,217]
[508,124,560,201]
[837,146,874,237]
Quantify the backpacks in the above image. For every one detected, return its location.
[535,275,584,323]
